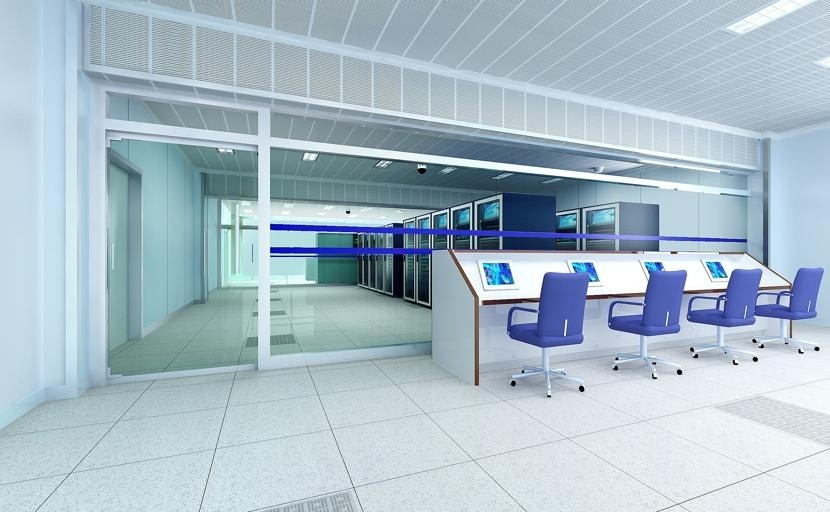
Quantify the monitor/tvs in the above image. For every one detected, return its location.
[477,259,520,291]
[566,259,604,287]
[639,259,667,280]
[700,258,730,281]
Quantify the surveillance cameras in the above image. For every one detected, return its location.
[417,164,427,174]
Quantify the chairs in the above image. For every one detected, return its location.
[507,271,589,397]
[752,267,824,354]
[608,270,687,378]
[686,269,762,365]
